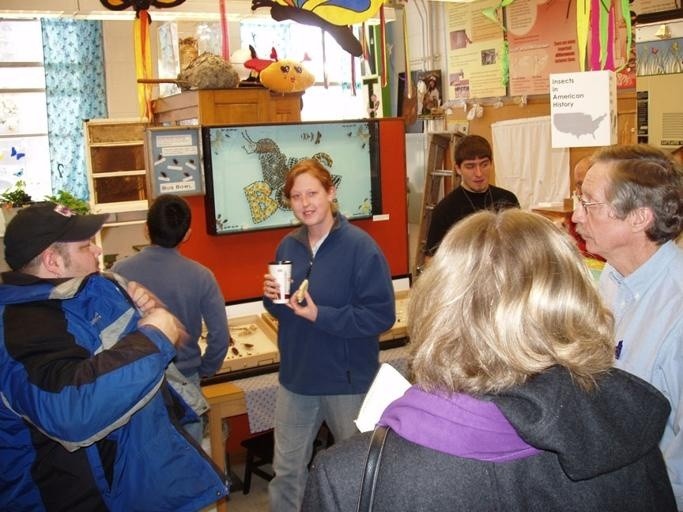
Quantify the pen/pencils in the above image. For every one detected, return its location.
[615,341,623,360]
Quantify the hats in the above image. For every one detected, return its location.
[2,200,110,272]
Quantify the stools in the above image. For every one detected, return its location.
[241,431,322,494]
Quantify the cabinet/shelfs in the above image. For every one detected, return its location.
[81,88,411,304]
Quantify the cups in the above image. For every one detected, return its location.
[267,261,292,305]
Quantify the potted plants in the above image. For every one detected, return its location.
[0,188,33,231]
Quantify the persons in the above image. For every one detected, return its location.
[295,209,683,511]
[0,203,231,510]
[1,201,179,510]
[112,193,230,448]
[573,153,595,202]
[423,133,521,268]
[263,159,396,510]
[572,139,683,509]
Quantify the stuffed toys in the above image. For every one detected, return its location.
[257,59,317,94]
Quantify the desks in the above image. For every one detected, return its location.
[201,349,413,512]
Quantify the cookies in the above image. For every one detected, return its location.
[238,324,258,336]
[297,278,309,303]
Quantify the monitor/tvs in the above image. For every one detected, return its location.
[202,120,382,236]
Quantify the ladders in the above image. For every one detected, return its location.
[412,130,466,288]
